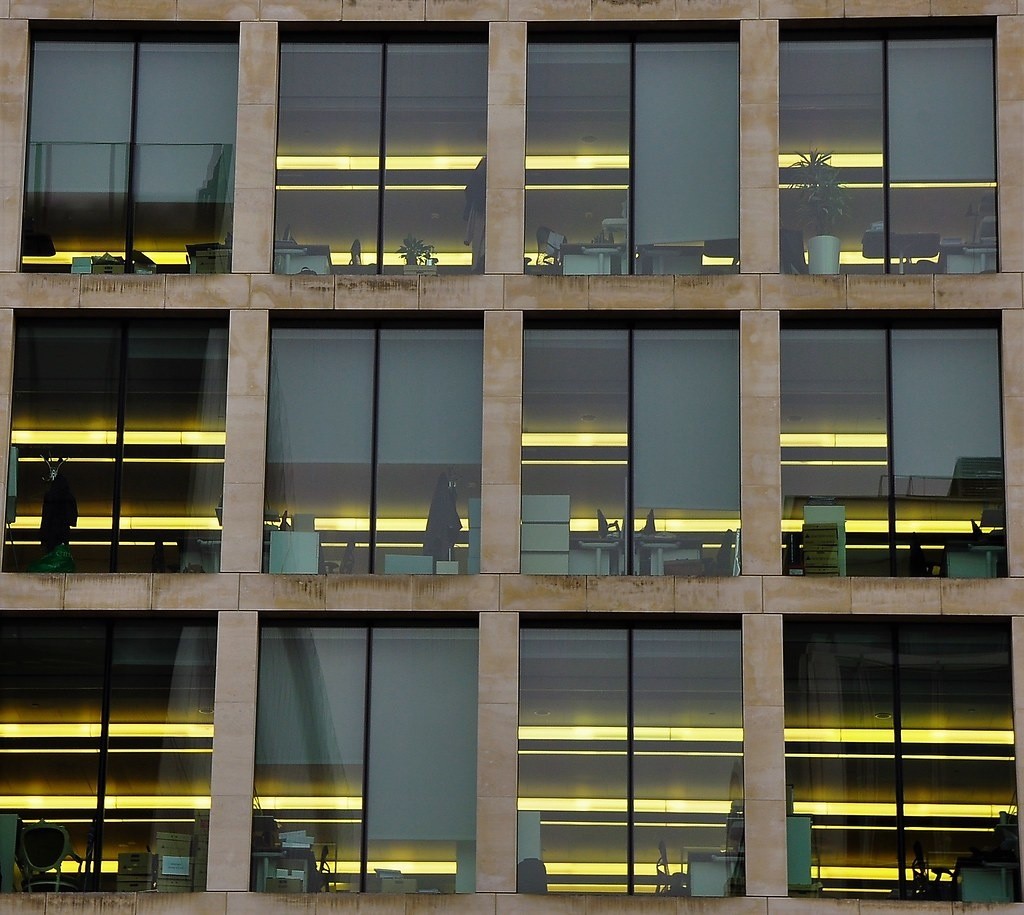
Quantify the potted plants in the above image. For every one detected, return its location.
[394,232,435,265]
[782,147,853,274]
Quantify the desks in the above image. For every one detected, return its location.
[686,851,745,897]
[177,529,270,574]
[559,243,705,275]
[186,244,331,274]
[269,530,326,575]
[864,233,941,274]
[952,855,1020,903]
[945,541,1007,577]
[119,528,204,574]
[569,537,703,576]
[93,264,125,274]
[385,554,435,575]
[939,244,998,274]
[251,848,317,894]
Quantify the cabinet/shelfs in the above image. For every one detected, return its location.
[517,810,543,862]
[454,839,477,894]
[787,816,813,885]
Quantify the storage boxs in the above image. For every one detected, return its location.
[804,567,841,577]
[803,546,839,567]
[117,851,158,875]
[157,879,193,894]
[436,561,460,575]
[467,497,482,527]
[521,493,572,523]
[193,858,207,880]
[118,875,157,892]
[789,885,818,898]
[787,783,794,815]
[191,834,208,857]
[156,832,193,857]
[290,513,315,532]
[158,856,194,880]
[468,557,481,574]
[193,809,210,835]
[403,265,438,276]
[194,880,206,892]
[521,523,570,551]
[381,879,419,893]
[195,249,232,272]
[468,527,481,557]
[803,523,839,545]
[521,551,568,576]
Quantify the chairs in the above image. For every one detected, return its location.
[702,528,733,577]
[911,532,945,577]
[71,256,92,275]
[18,817,84,892]
[656,841,687,896]
[322,537,356,574]
[338,240,363,275]
[311,846,331,894]
[910,839,958,902]
